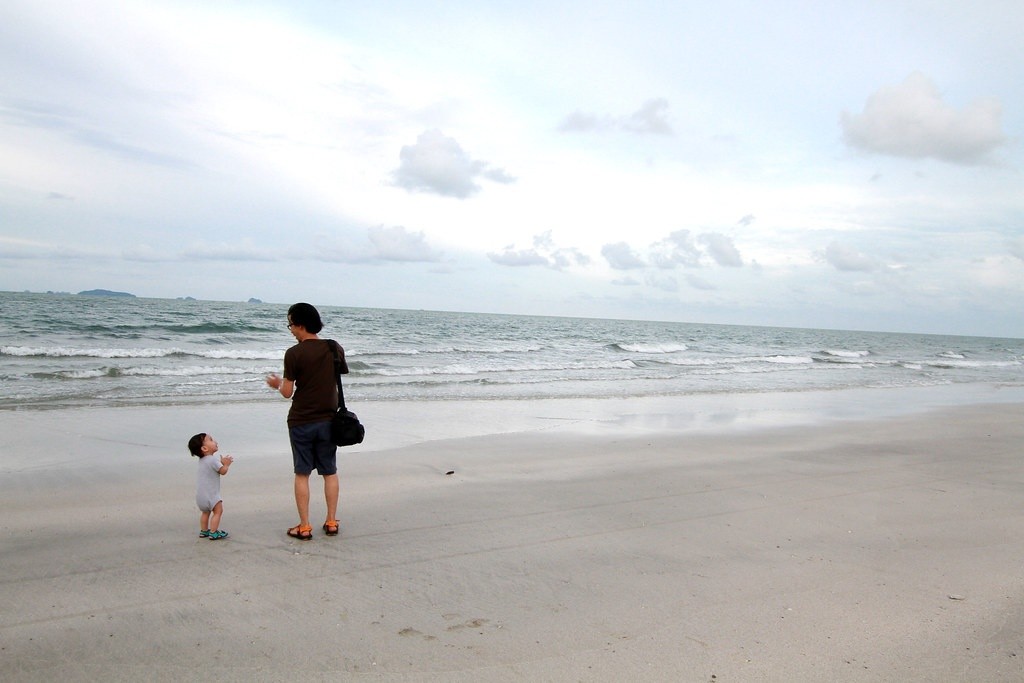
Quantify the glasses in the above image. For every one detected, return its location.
[287,323,294,330]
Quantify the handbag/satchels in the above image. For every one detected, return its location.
[336,408,365,447]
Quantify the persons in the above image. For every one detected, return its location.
[188,432,234,540]
[264,302,349,541]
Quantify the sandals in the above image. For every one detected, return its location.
[287,524,313,540]
[323,520,340,535]
[199,529,210,537]
[209,530,228,540]
